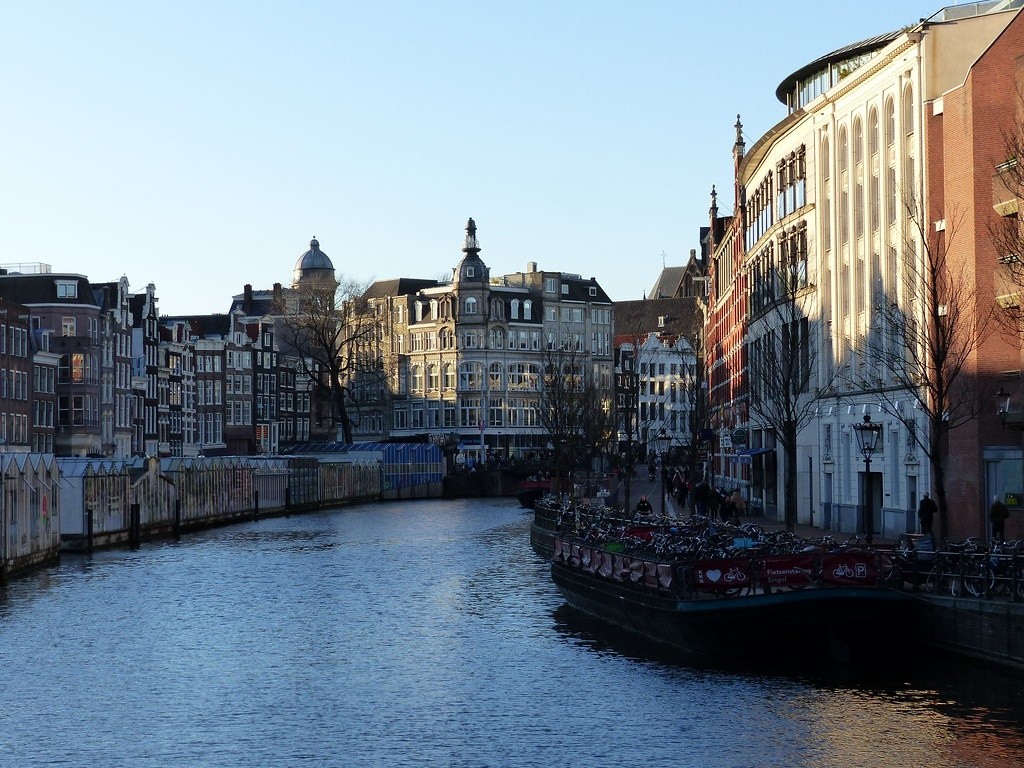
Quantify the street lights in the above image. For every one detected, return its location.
[851,411,882,545]
[656,428,673,504]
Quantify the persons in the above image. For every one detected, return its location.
[635,494,653,514]
[647,462,655,478]
[988,495,1010,538]
[918,491,938,536]
[660,464,742,523]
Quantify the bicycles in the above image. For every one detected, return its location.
[533,489,1023,603]
